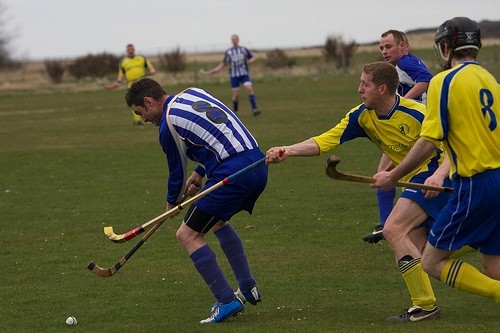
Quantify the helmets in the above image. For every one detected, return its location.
[434,16,482,69]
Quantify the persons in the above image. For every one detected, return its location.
[205,34,261,116]
[371,15,500,307]
[362,29,433,245]
[125,78,270,324]
[264,61,458,325]
[113,43,158,128]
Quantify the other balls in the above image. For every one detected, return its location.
[65,317,78,326]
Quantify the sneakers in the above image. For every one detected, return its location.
[234,286,261,305]
[362,223,388,243]
[201,295,245,325]
[386,304,441,324]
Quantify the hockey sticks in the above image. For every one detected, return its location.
[200,62,247,76]
[87,182,201,278]
[326,155,455,194]
[103,147,285,243]
[104,73,156,89]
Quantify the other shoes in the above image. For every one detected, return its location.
[252,108,261,115]
[133,121,143,126]
